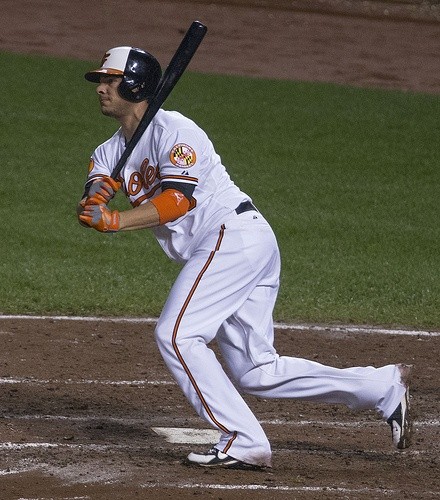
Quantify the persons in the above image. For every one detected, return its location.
[75,46,417,467]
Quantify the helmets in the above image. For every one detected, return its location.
[85,46,162,102]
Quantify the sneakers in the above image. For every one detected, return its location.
[183,447,267,471]
[385,385,412,448]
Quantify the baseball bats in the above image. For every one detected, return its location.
[110,20,209,182]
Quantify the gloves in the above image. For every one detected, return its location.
[85,176,122,204]
[79,204,119,231]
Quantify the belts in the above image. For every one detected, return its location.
[235,201,257,215]
